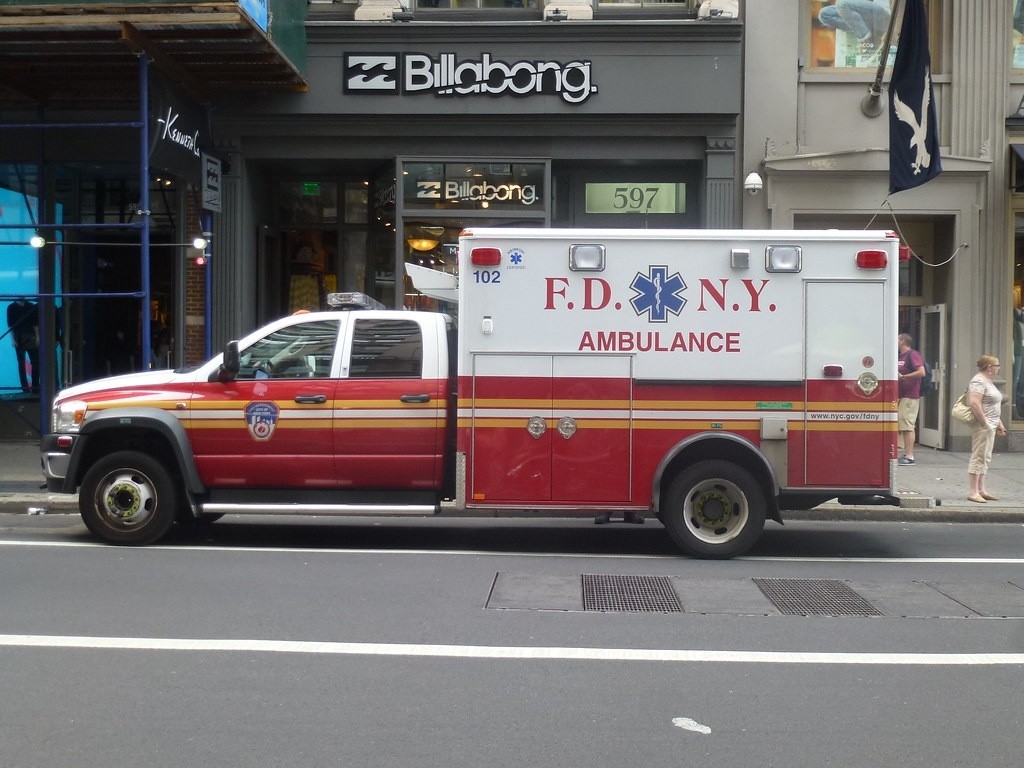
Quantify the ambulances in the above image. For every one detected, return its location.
[38,226,911,561]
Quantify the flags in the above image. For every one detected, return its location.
[889,0,942,195]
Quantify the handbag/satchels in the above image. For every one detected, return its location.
[952,376,987,424]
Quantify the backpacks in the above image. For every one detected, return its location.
[907,350,932,396]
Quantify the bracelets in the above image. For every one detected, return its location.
[902,375,906,379]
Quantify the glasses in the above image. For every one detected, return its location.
[992,365,1000,367]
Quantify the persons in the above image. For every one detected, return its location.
[150,299,167,357]
[898,334,926,465]
[965,355,1007,503]
[819,0,892,66]
[7,297,40,394]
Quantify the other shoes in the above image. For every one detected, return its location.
[897,455,915,466]
[982,494,998,500]
[967,495,986,503]
